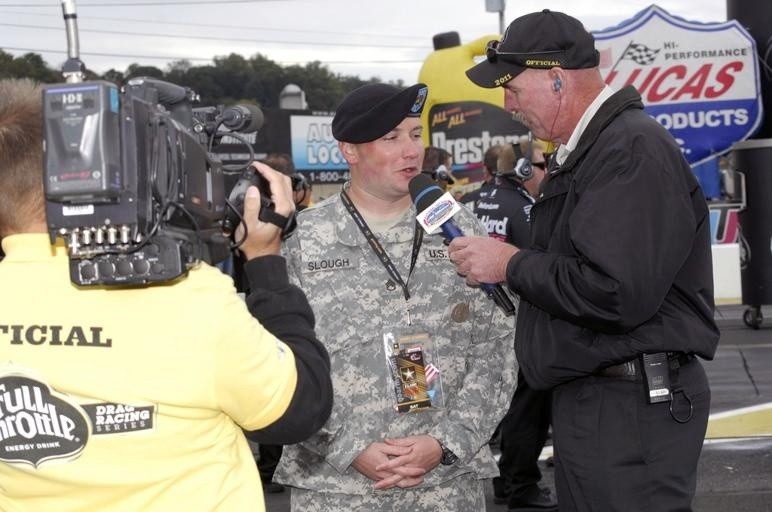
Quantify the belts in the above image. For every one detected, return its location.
[593,351,694,378]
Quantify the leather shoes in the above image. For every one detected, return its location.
[492,475,560,511]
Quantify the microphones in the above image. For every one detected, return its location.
[409,175,517,317]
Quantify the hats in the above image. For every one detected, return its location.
[332,83,428,144]
[465,9,599,88]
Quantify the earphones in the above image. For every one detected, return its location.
[554,80,562,92]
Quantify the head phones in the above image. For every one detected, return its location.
[497,139,533,181]
[421,149,454,184]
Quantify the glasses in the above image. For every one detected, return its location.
[487,40,570,65]
[531,160,547,171]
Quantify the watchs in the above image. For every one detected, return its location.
[438,440,458,465]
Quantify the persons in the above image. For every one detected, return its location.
[459,140,559,512]
[450,9,720,512]
[272,83,518,512]
[257,146,505,241]
[0,78,332,512]
